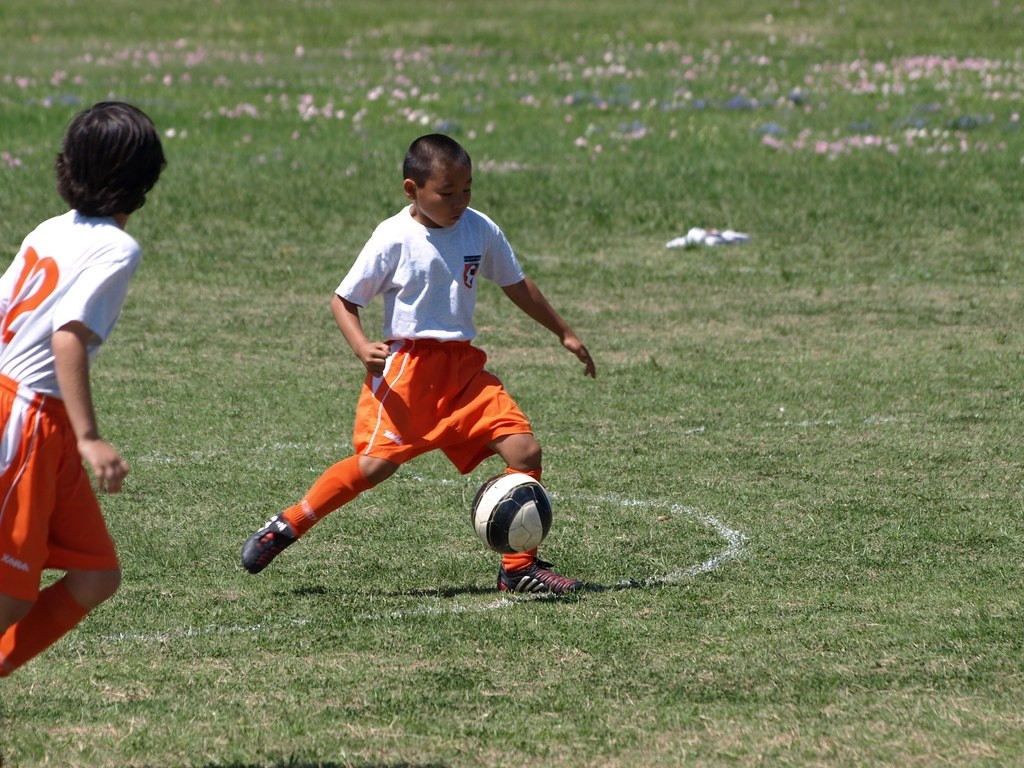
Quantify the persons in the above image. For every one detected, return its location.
[0,100,170,676]
[241,133,597,594]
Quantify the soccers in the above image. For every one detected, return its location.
[470,472,553,554]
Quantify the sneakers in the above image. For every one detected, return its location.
[241,513,296,575]
[497,557,582,593]
